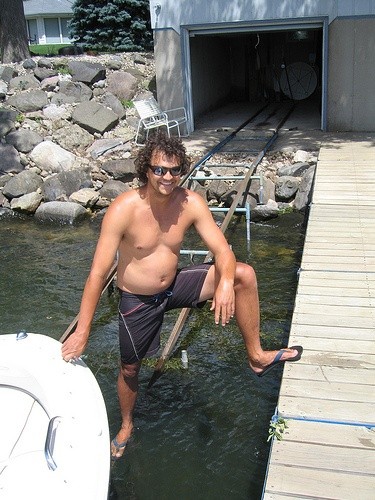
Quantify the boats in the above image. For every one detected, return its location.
[0,332,111,500]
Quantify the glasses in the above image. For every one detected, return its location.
[148,164,183,176]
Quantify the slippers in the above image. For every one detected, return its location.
[257,345,303,377]
[111,436,128,460]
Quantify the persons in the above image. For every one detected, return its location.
[61,133,302,460]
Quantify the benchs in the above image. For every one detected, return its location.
[132,98,190,148]
